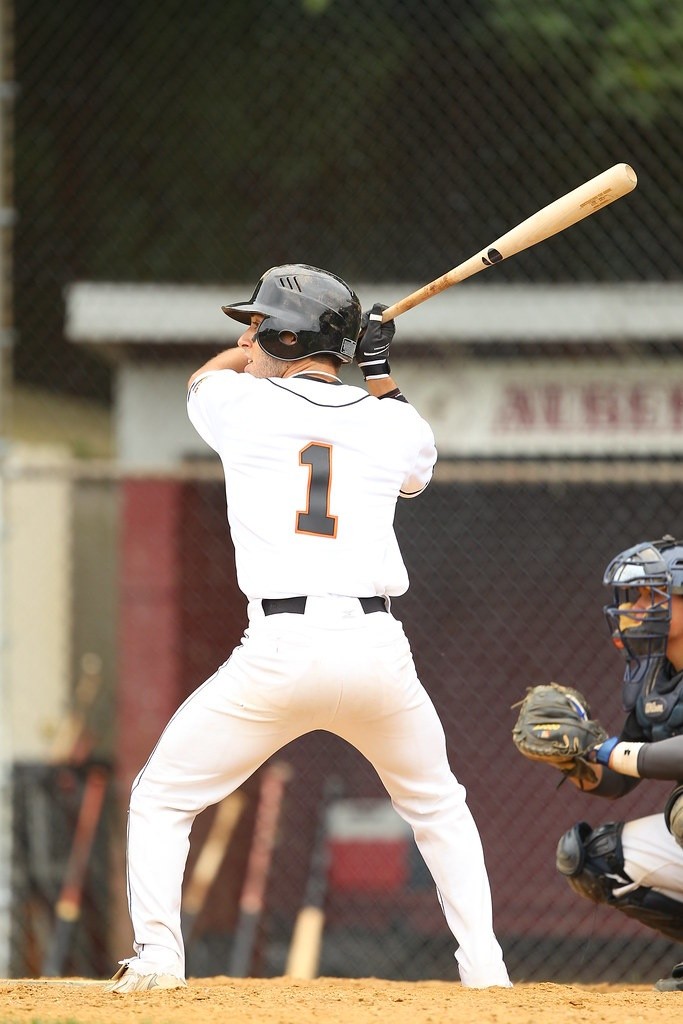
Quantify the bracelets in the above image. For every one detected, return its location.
[612,741,643,779]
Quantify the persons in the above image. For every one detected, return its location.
[512,533,683,992]
[115,264,511,999]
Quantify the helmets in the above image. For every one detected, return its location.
[603,533,683,596]
[221,264,362,365]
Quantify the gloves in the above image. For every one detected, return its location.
[355,303,395,381]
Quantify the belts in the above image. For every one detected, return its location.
[262,597,387,616]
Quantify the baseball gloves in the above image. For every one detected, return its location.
[513,680,614,775]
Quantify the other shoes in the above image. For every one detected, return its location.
[657,961,683,992]
[110,965,187,993]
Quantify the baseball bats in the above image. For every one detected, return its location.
[42,755,114,977]
[230,764,289,977]
[351,163,639,345]
[181,784,250,943]
[287,777,347,979]
